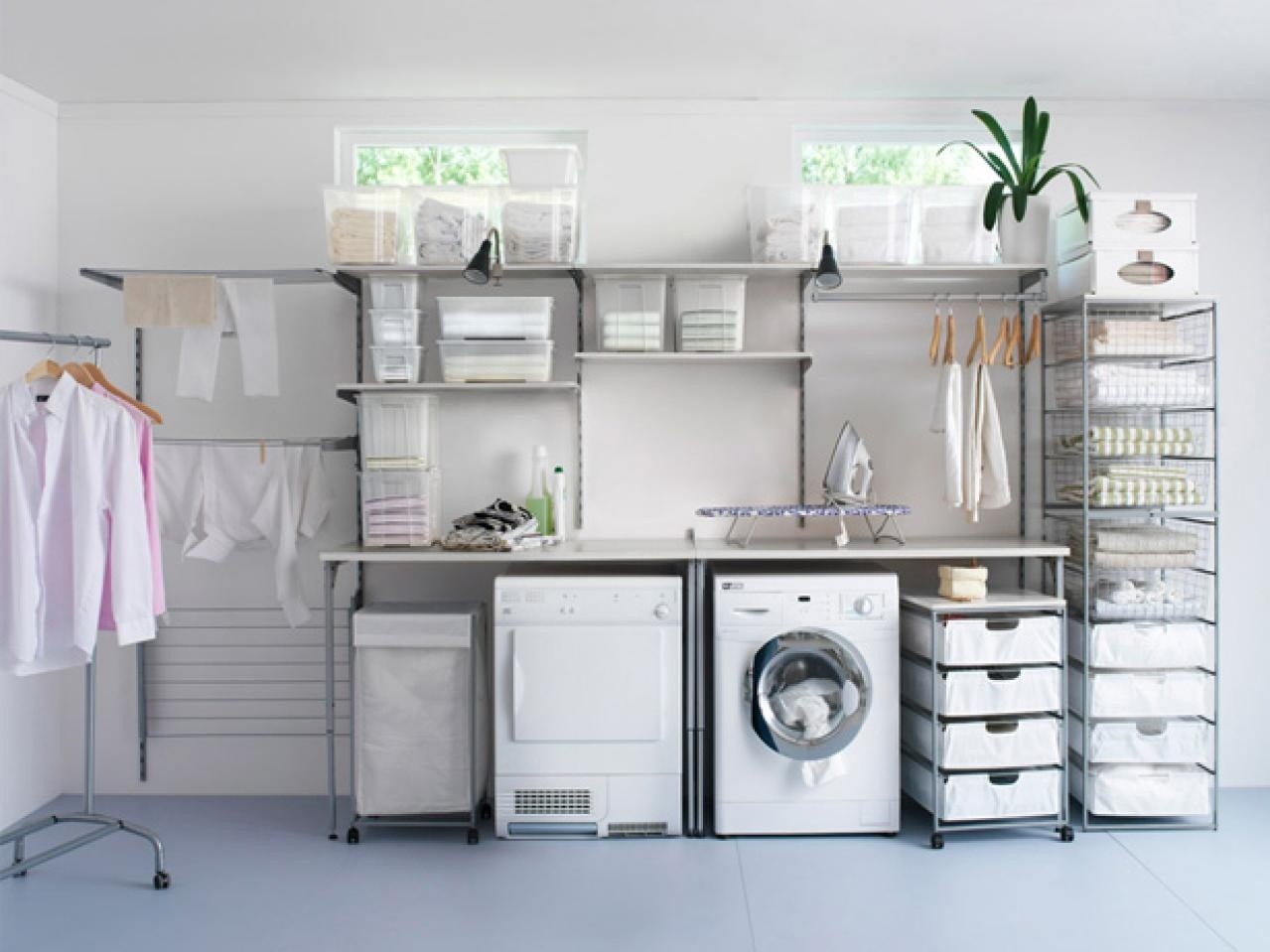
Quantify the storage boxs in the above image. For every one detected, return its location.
[405,184,492,267]
[590,272,668,352]
[367,272,425,310]
[1057,239,1201,295]
[316,182,404,266]
[669,273,750,352]
[917,183,999,266]
[434,338,556,384]
[830,184,916,265]
[355,471,442,550]
[353,391,440,472]
[492,181,583,268]
[1054,189,1199,257]
[433,294,556,340]
[369,346,425,384]
[366,309,429,345]
[745,179,831,263]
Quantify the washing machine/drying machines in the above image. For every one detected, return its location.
[711,563,904,836]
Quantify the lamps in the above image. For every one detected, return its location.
[816,232,844,292]
[461,228,501,287]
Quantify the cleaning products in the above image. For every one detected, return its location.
[525,445,567,541]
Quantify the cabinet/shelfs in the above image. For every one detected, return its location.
[327,262,1049,410]
[1039,291,1220,835]
[904,588,1077,850]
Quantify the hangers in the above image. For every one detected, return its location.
[1023,291,1040,366]
[964,292,991,367]
[22,331,64,384]
[62,333,95,389]
[928,291,943,366]
[943,293,956,365]
[1002,293,1026,370]
[79,334,164,424]
[983,293,1011,368]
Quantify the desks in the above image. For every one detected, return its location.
[318,534,1074,844]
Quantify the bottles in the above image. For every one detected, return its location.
[1116,200,1169,233]
[553,464,566,541]
[524,444,554,536]
[1117,250,1174,284]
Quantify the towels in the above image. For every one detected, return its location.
[1058,464,1194,507]
[1053,319,1198,358]
[1055,364,1207,407]
[1060,426,1198,454]
[443,497,540,551]
[933,361,1014,526]
[1070,577,1178,621]
[1069,526,1200,567]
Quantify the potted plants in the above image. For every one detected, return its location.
[936,92,1102,265]
